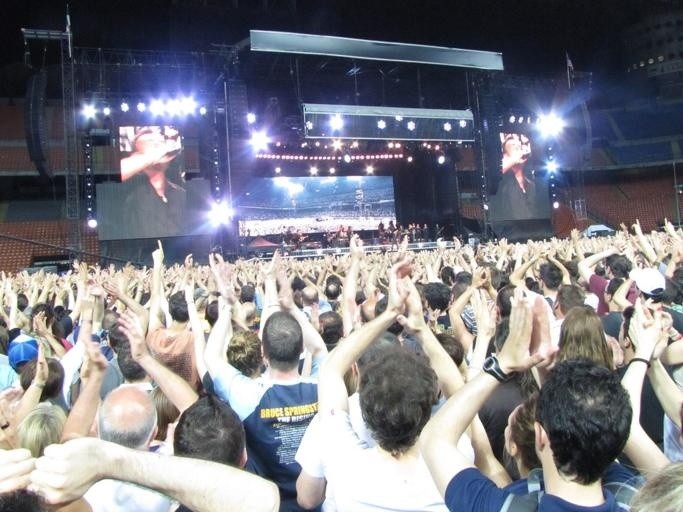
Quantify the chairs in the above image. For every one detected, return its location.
[180,122,202,172]
[1,106,117,174]
[461,184,482,223]
[457,146,477,171]
[0,220,98,276]
[553,103,681,238]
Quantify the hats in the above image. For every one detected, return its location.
[9,335,38,369]
[629,268,666,295]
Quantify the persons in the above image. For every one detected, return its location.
[503,135,535,218]
[121,126,186,233]
[0,216,682,512]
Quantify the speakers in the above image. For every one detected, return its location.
[23,265,57,276]
[23,73,50,162]
[578,100,593,161]
